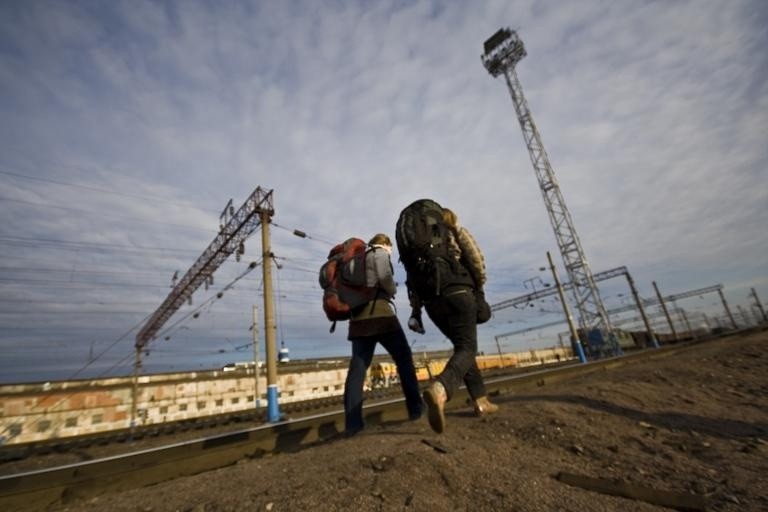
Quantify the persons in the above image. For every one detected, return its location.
[407,208,500,434]
[342,233,423,439]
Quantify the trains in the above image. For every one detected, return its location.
[571,328,693,356]
[370,356,517,389]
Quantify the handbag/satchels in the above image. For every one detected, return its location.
[474,287,490,323]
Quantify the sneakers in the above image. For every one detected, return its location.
[423,380,448,433]
[473,396,498,417]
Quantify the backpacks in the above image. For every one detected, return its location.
[396,199,461,301]
[319,238,377,321]
